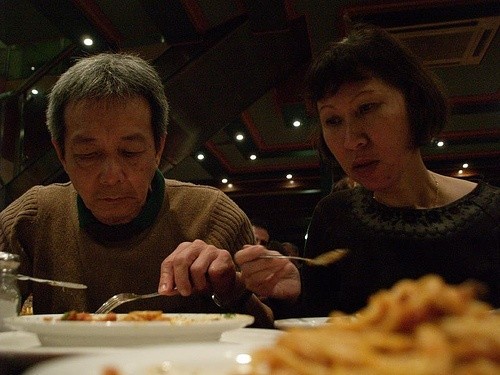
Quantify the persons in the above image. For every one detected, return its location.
[273,238,299,268]
[231,28,500,321]
[0,51,278,327]
[247,218,271,251]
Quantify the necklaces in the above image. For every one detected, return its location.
[426,168,440,210]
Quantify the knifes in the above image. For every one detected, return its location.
[18,273,89,289]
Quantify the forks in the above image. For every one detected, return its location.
[94,287,194,314]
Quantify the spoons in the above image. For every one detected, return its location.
[257,249,347,266]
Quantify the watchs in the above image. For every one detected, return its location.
[212,285,245,313]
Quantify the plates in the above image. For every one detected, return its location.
[274,312,361,333]
[2,305,256,346]
[0,326,292,375]
